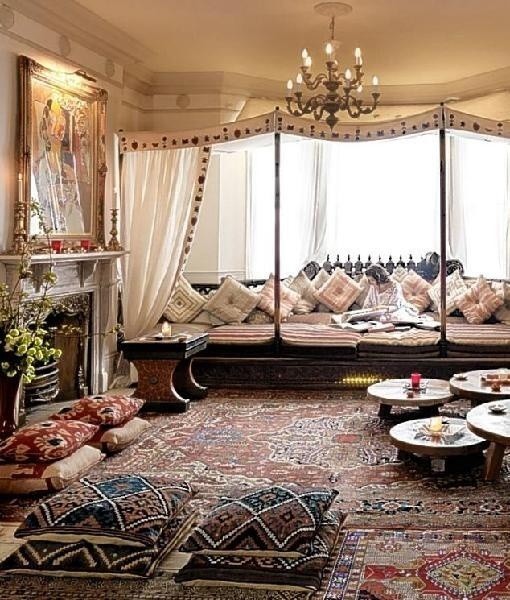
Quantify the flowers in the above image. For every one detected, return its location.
[0,197,125,384]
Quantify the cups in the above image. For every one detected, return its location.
[430,416,442,431]
[410,373,421,386]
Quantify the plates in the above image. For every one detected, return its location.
[453,373,468,379]
[403,382,426,389]
[487,404,507,412]
[413,423,466,436]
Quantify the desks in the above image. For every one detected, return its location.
[367,367,510,480]
[1,248,130,393]
[120,331,209,414]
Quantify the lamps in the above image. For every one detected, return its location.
[284,1,381,133]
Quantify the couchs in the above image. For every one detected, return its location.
[159,252,509,364]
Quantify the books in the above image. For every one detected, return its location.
[366,321,395,333]
[331,307,386,323]
[327,320,381,332]
[385,325,412,334]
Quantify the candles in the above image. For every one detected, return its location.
[112,133,120,209]
[16,172,23,202]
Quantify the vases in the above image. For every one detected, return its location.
[0,345,23,442]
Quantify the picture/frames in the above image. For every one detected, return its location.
[19,48,108,250]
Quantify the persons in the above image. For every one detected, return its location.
[32,100,85,235]
[359,263,419,326]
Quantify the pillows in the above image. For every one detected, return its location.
[50,393,152,452]
[164,270,501,324]
[177,485,348,591]
[3,420,107,493]
[2,474,200,581]
